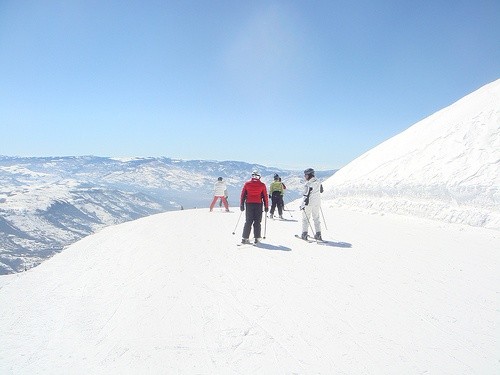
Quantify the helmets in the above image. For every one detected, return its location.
[217,176,223,181]
[304,167,315,176]
[251,170,261,179]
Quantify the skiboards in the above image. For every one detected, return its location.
[206,208,235,214]
[267,215,288,221]
[236,236,263,246]
[295,234,329,244]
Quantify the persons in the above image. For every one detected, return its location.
[239,170,269,244]
[268,172,286,220]
[299,168,324,240]
[210,176,233,212]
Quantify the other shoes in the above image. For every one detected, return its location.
[314,233,322,240]
[254,238,259,243]
[279,215,283,219]
[241,238,250,244]
[301,232,307,240]
[269,213,273,219]
